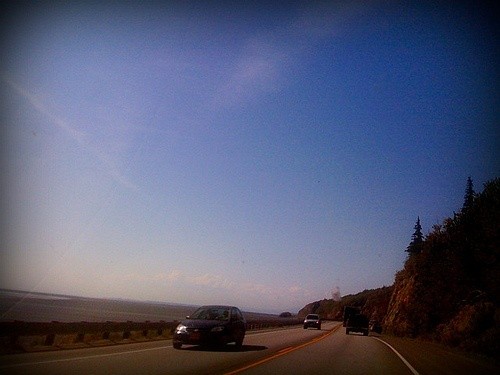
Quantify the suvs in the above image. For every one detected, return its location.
[346,314,370,336]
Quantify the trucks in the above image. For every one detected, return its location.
[342,303,361,327]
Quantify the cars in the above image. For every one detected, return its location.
[303,314,322,330]
[172,305,246,351]
